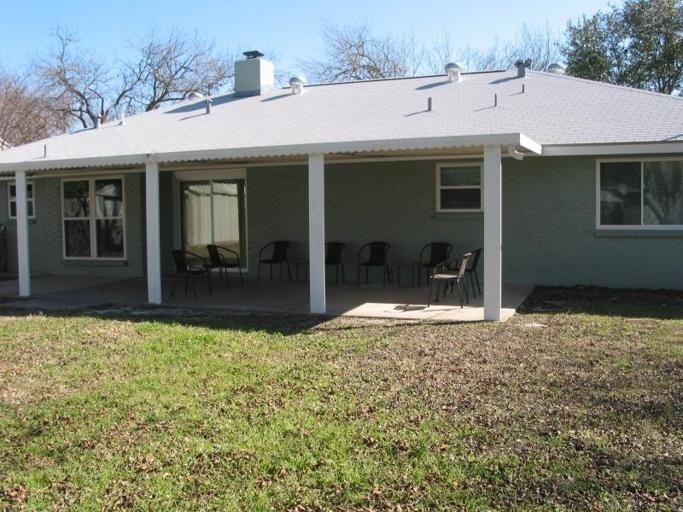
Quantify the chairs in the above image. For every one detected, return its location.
[206,244,244,289]
[356,241,392,288]
[413,241,483,308]
[257,241,292,284]
[325,242,346,289]
[170,249,213,298]
